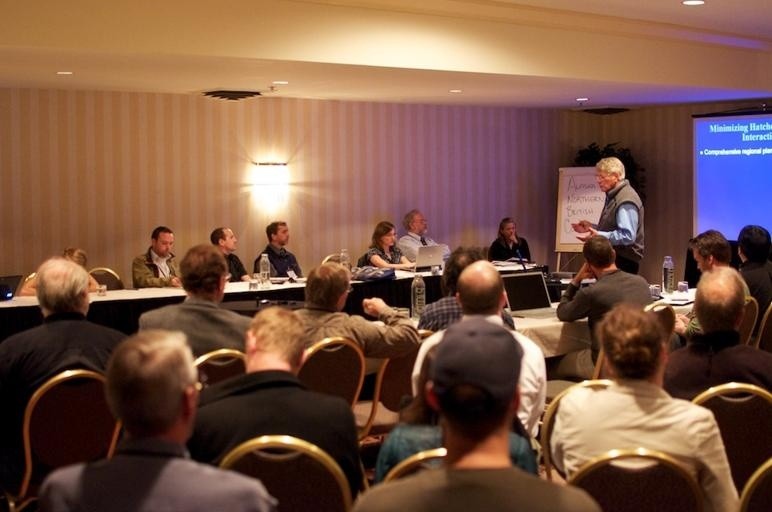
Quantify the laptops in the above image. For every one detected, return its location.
[0,275,23,301]
[500,271,557,319]
[400,245,442,272]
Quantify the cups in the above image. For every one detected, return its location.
[678,281,688,292]
[96,284,107,297]
[652,284,661,296]
[249,280,258,290]
[430,265,440,275]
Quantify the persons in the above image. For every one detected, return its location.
[283,264,421,382]
[210,227,252,282]
[669,227,772,352]
[554,236,647,378]
[42,325,278,511]
[547,305,741,510]
[131,225,182,288]
[0,256,128,419]
[185,305,360,493]
[339,323,594,511]
[412,246,547,433]
[253,210,530,281]
[139,244,251,359]
[20,248,99,296]
[378,345,538,478]
[570,157,645,276]
[662,269,768,397]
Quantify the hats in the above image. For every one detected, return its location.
[430,317,524,411]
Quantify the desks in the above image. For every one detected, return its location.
[547,280,589,302]
[1,266,549,336]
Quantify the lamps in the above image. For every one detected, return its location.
[249,161,290,188]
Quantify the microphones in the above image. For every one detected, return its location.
[516,249,527,273]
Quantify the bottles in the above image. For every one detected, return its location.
[260,254,270,289]
[340,249,350,271]
[662,256,673,295]
[411,274,425,321]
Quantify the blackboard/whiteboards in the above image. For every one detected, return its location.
[554,167,606,252]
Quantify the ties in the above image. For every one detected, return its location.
[421,237,426,246]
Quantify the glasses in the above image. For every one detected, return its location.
[224,272,232,281]
[194,371,209,392]
[345,286,354,293]
[414,219,427,224]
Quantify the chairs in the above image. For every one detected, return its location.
[320,253,353,271]
